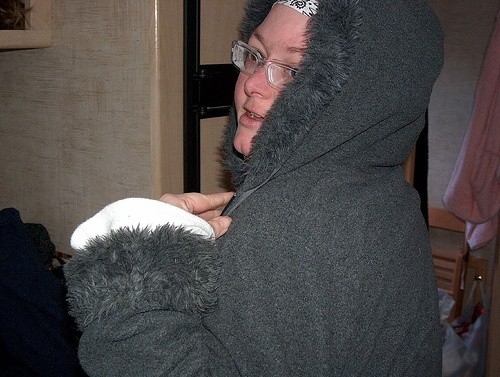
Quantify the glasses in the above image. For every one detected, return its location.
[230,39,299,90]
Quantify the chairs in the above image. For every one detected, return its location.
[427,207,492,334]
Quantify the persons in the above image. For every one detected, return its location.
[63,0,446,377]
